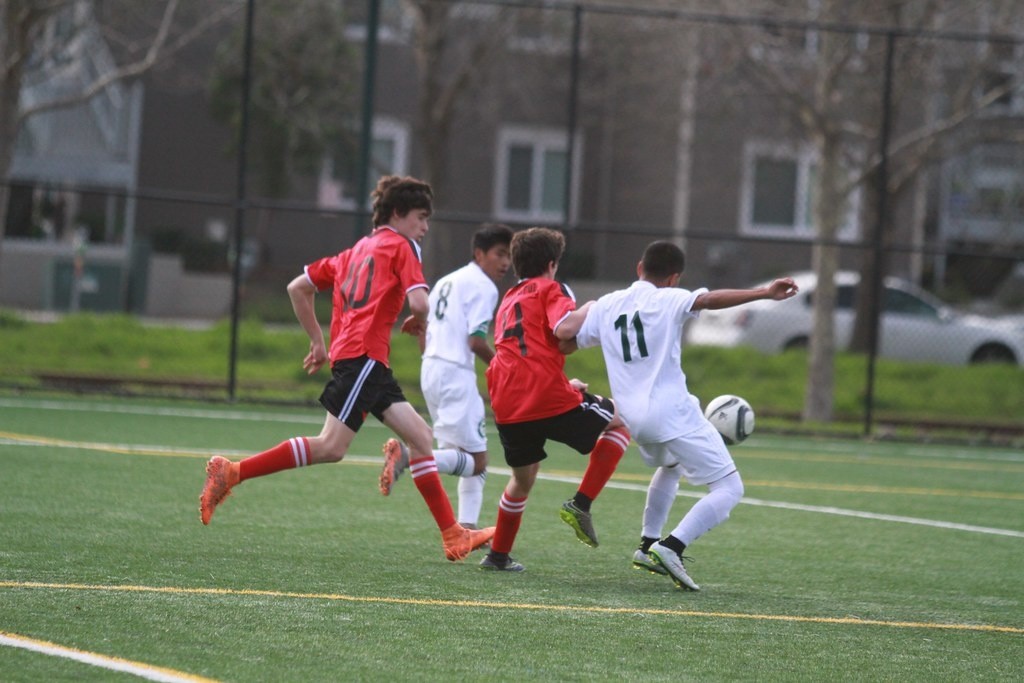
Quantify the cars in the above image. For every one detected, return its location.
[688,269,1022,368]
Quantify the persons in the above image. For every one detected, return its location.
[478,228,628,573]
[559,240,800,591]
[380,222,514,547]
[198,174,492,562]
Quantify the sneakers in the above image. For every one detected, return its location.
[444,529,497,561]
[559,500,599,547]
[647,541,700,591]
[380,438,408,496]
[632,542,670,576]
[479,548,526,571]
[198,455,233,525]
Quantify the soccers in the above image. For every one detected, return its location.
[704,394,756,446]
